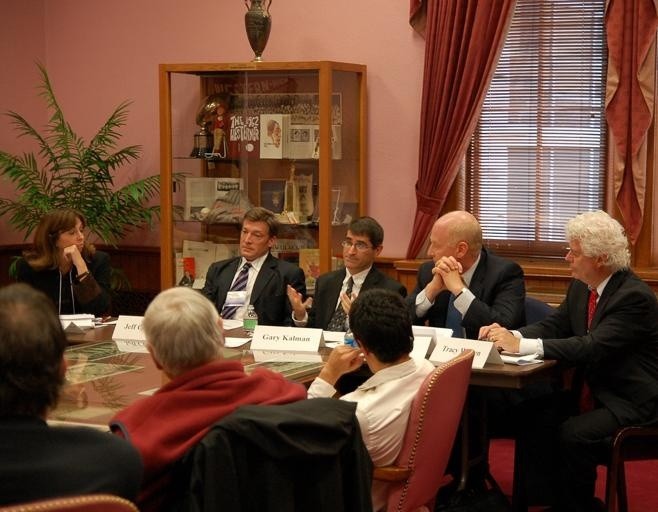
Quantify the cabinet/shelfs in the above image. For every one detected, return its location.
[160,60,367,288]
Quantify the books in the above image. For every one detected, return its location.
[227,112,342,159]
[175,240,241,290]
[185,177,249,221]
[260,164,348,223]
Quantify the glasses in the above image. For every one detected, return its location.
[341,241,372,252]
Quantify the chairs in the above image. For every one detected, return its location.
[599,419,658,512]
[372,348,475,512]
[139,399,373,512]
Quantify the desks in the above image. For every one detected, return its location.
[42,313,556,504]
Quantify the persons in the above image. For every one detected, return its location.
[404,210,525,512]
[479,210,658,511]
[201,208,307,327]
[288,216,408,333]
[307,290,436,511]
[0,284,142,512]
[16,210,121,318]
[109,287,307,512]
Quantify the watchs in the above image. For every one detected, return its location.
[453,285,468,301]
[76,271,89,280]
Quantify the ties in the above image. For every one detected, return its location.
[327,276,354,332]
[577,289,597,417]
[446,293,464,338]
[220,263,252,319]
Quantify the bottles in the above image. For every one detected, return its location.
[339,323,359,353]
[243,306,259,335]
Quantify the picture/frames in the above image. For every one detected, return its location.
[257,176,287,214]
